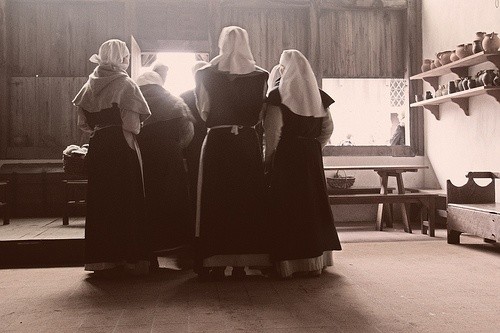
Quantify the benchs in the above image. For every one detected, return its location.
[329,193,437,237]
[447,171,500,247]
[325,188,396,227]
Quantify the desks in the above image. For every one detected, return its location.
[323,165,429,234]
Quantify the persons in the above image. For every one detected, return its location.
[134,71,195,278]
[180,62,210,275]
[193,26,270,283]
[266,49,342,279]
[71,39,151,276]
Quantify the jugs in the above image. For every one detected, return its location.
[425,69,500,100]
[421,32,500,72]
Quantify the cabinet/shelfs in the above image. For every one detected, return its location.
[410,50,500,120]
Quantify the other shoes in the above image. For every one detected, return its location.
[232,270,246,281]
[210,270,225,280]
